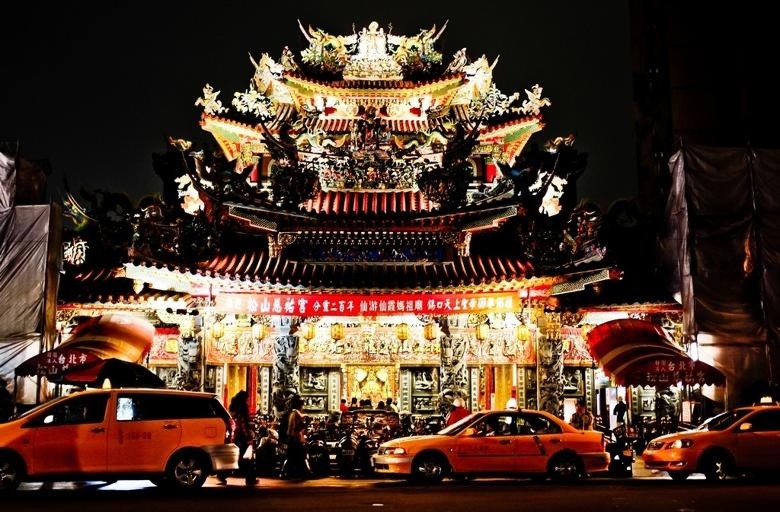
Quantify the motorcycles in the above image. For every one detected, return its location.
[254,419,381,477]
[603,426,638,480]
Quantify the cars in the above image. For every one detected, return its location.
[642,403,780,484]
[0,387,241,493]
[370,409,611,487]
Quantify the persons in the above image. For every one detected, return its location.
[475,417,552,436]
[217,390,470,486]
[571,399,593,429]
[612,397,630,427]
[651,390,681,423]
